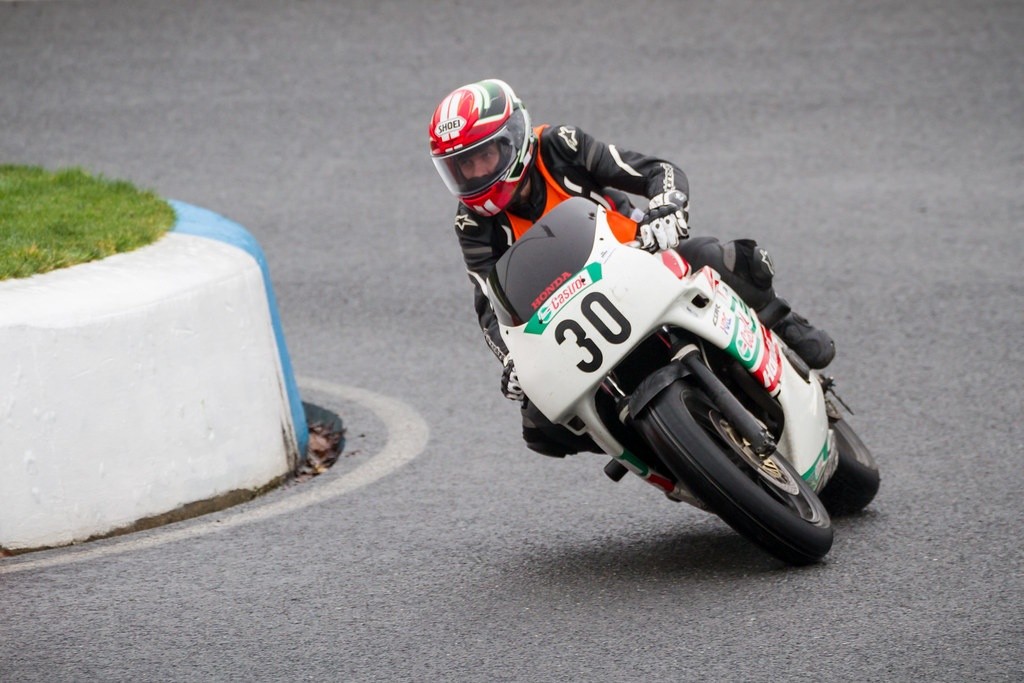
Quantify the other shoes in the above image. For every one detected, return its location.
[774,311,835,370]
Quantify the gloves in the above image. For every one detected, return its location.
[639,190,689,254]
[498,354,528,403]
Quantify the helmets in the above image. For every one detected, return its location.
[427,79,533,218]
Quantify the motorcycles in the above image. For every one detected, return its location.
[480,196,881,566]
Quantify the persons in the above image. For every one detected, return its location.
[426,77,838,459]
